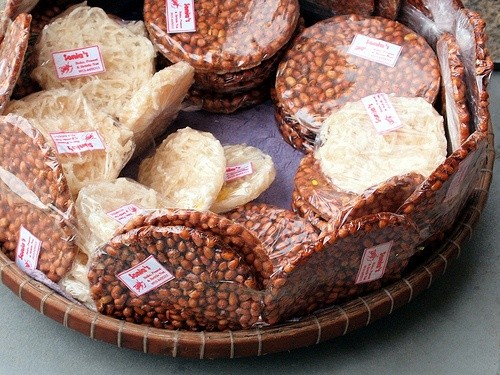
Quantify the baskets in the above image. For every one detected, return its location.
[0,0,495,360]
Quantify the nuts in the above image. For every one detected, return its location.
[0,0,494,332]
